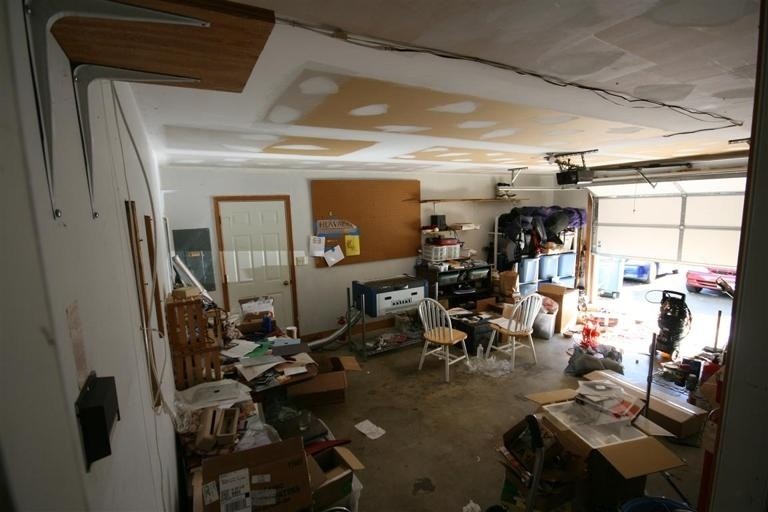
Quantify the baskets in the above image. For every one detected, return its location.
[422,244,460,262]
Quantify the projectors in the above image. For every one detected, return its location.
[556,170,593,185]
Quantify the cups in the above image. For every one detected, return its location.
[287,327,297,340]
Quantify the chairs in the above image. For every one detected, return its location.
[418,298,473,383]
[484,293,544,372]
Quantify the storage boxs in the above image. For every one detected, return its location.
[517,252,576,298]
[537,283,579,335]
[585,369,707,438]
[475,297,497,313]
[306,447,366,512]
[438,296,449,310]
[191,436,312,512]
[284,354,362,409]
[525,391,686,480]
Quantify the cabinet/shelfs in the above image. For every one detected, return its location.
[414,265,493,311]
[487,211,583,298]
[347,282,438,359]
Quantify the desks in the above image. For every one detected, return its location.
[177,324,319,414]
[439,312,505,356]
[489,302,516,345]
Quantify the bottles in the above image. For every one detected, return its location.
[662,364,696,390]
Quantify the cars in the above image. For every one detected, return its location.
[624,257,739,293]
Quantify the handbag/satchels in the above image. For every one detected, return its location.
[500,272,519,295]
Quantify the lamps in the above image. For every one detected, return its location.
[496,165,582,191]
